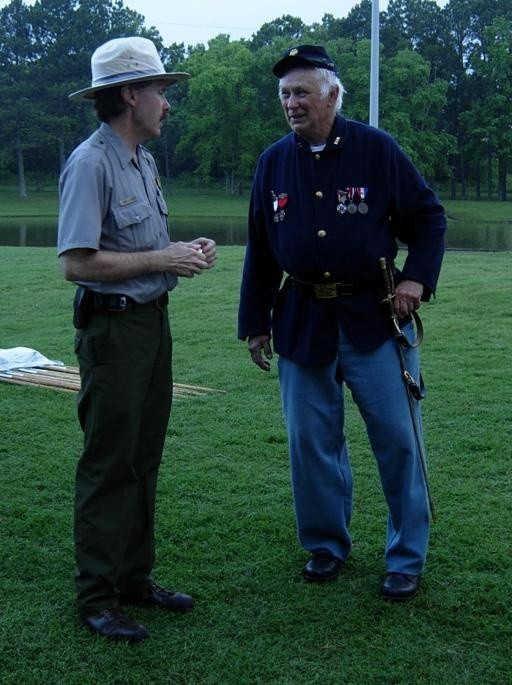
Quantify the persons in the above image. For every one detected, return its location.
[56,33,219,645]
[236,44,449,600]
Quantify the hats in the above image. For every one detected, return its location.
[273,45,336,78]
[67,36,191,103]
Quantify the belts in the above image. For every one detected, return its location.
[89,291,169,312]
[289,275,368,299]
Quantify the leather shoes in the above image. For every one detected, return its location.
[381,571,418,599]
[85,607,147,640]
[303,553,342,581]
[141,581,194,610]
